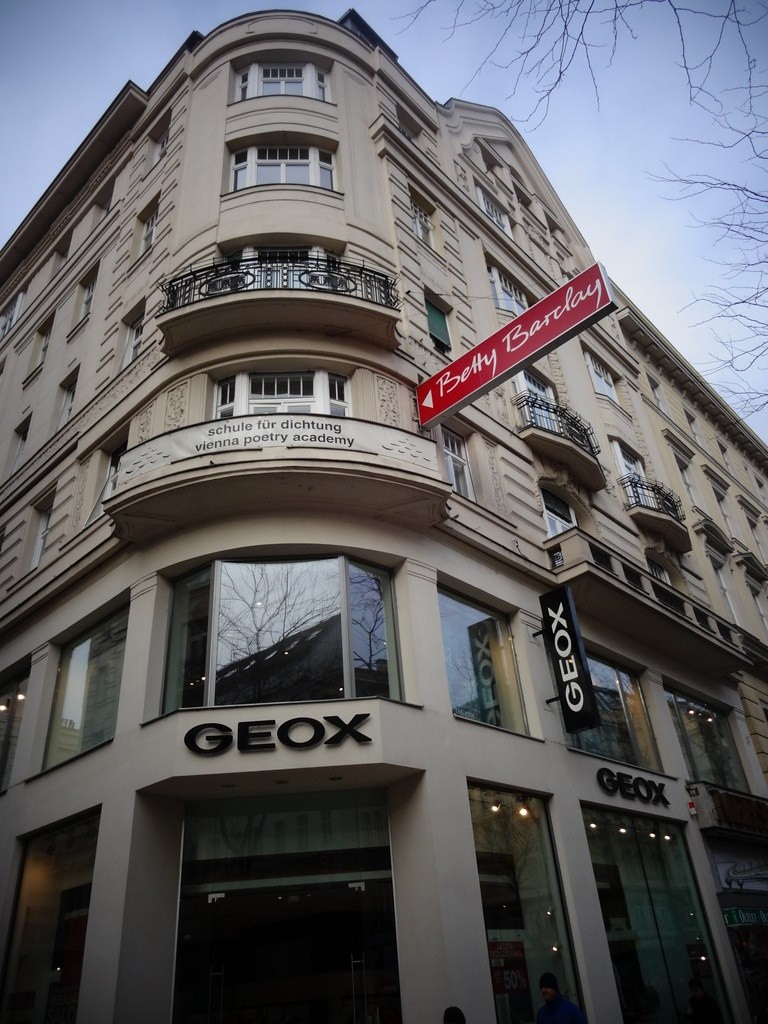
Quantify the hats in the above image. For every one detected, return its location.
[540,971,558,992]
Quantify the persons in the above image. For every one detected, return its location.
[443,1007,466,1024]
[685,979,724,1024]
[536,972,587,1024]
